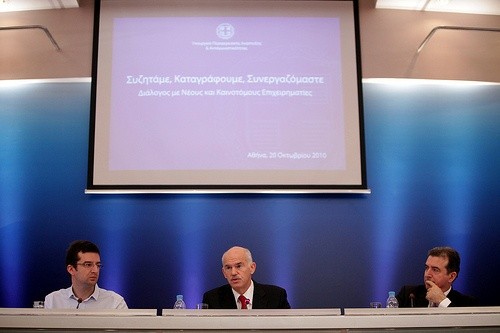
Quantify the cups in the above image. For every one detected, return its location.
[197,303,208,309]
[34,301,44,308]
[370,302,381,308]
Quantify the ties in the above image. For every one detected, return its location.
[238,295,250,309]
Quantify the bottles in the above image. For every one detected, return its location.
[173,295,186,309]
[386,291,399,308]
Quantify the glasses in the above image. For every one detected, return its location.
[70,262,104,270]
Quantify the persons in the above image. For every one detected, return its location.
[44,240,129,309]
[202,246,291,309]
[395,247,468,308]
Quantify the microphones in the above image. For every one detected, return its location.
[77,298,82,309]
[246,301,249,304]
[409,294,414,308]
